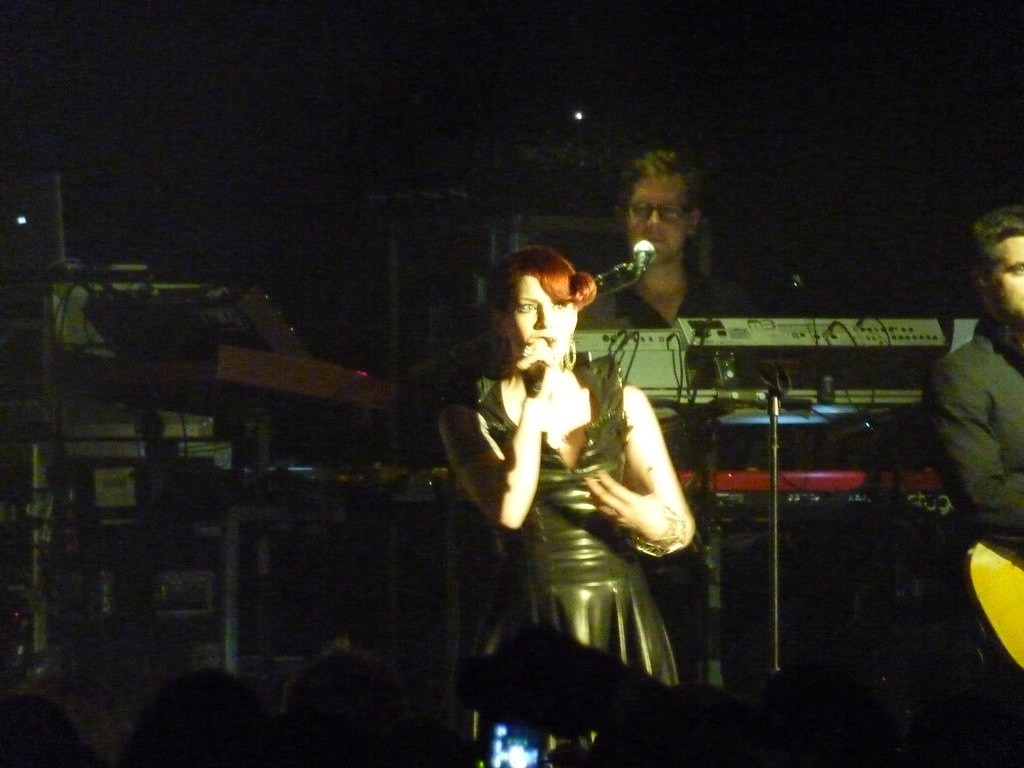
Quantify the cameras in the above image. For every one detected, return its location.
[473,701,548,768]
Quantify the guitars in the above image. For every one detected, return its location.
[961,534,1024,674]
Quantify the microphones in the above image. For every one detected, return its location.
[526,337,551,399]
[632,239,655,276]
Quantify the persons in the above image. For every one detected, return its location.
[438,150,736,768]
[927,205,1023,547]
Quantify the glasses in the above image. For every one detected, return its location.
[629,200,691,223]
